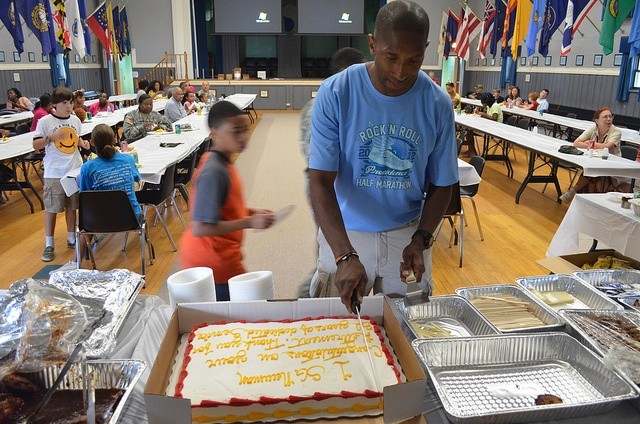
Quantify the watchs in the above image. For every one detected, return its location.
[412,229,433,249]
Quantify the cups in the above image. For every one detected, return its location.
[166,267,217,313]
[226,271,274,300]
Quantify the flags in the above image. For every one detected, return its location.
[0,1,132,63]
[437,1,640,60]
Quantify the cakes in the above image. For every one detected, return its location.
[174,316,403,423]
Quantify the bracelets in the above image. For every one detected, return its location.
[334,249,360,265]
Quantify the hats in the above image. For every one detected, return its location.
[558,145,583,155]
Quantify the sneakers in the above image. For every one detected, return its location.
[559,187,576,202]
[42,246,54,261]
[68,240,77,248]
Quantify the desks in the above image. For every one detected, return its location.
[451,107,640,203]
[0,88,138,134]
[0,97,176,214]
[546,185,638,266]
[457,153,482,196]
[460,98,640,149]
[103,93,257,224]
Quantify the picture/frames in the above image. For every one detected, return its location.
[84,55,89,63]
[532,56,538,66]
[13,72,20,82]
[631,69,640,90]
[0,51,5,62]
[42,53,48,62]
[490,58,496,65]
[92,54,97,63]
[260,90,269,98]
[575,55,584,65]
[520,57,526,65]
[482,59,487,66]
[545,56,551,66]
[311,90,318,98]
[500,57,503,65]
[524,73,531,83]
[28,52,35,62]
[559,56,566,66]
[593,54,603,66]
[614,54,623,66]
[75,54,80,62]
[13,51,21,61]
[474,59,479,66]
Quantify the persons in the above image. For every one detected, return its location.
[135,79,165,103]
[88,89,116,115]
[465,84,549,111]
[461,92,504,160]
[180,79,214,115]
[164,87,187,123]
[0,128,11,137]
[560,107,618,203]
[123,93,173,141]
[176,99,277,302]
[48,125,142,276]
[32,90,87,129]
[4,88,35,113]
[446,81,461,114]
[32,86,89,263]
[429,70,440,83]
[306,1,460,313]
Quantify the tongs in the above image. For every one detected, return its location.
[87,362,123,422]
[23,343,87,422]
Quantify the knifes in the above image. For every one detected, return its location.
[350,292,381,392]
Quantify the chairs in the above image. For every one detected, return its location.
[75,189,152,289]
[200,137,213,162]
[455,137,463,153]
[508,115,520,124]
[567,113,577,119]
[2,151,46,217]
[581,144,638,194]
[15,124,47,186]
[518,118,530,128]
[135,164,187,252]
[449,154,485,251]
[116,120,126,143]
[174,147,200,203]
[440,180,465,268]
